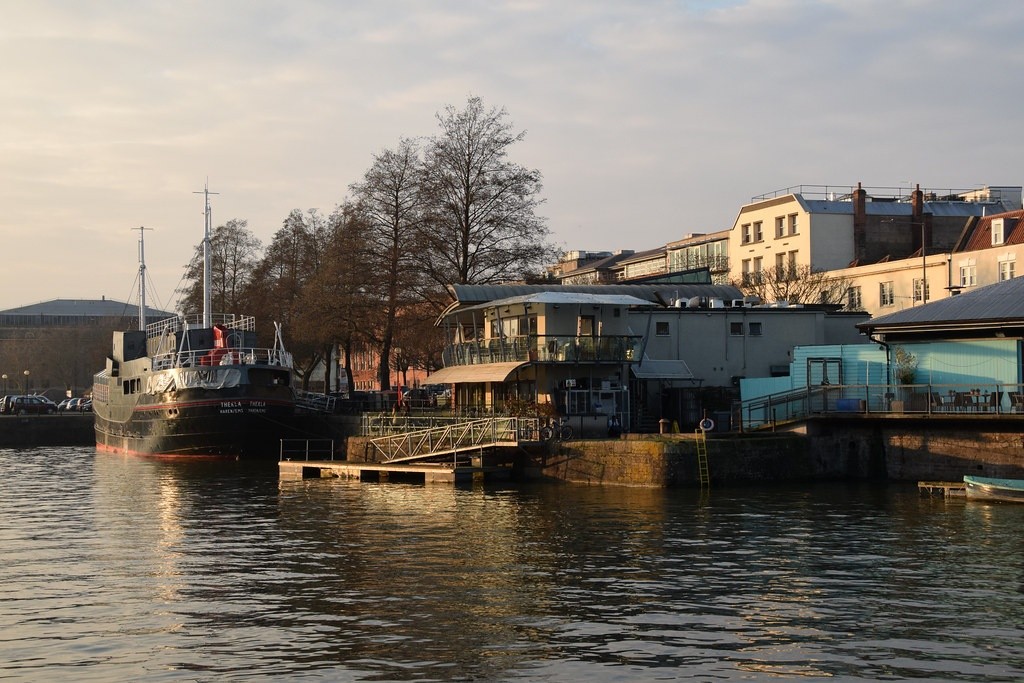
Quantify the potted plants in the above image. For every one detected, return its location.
[976,388,980,395]
[896,347,918,389]
[970,389,975,394]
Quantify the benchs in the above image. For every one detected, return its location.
[925,391,943,411]
[1007,392,1022,414]
[954,391,973,410]
[980,392,1004,412]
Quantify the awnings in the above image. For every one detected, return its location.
[631,359,695,380]
[423,362,530,385]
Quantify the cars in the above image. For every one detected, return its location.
[0,394,92,415]
[390,382,445,407]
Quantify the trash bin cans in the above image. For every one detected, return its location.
[837,398,862,410]
[715,411,732,432]
[859,400,866,411]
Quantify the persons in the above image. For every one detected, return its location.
[656,384,669,408]
[608,415,621,438]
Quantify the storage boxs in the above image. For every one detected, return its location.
[835,398,860,413]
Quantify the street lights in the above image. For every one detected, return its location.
[1,373,8,397]
[23,368,30,415]
[394,346,402,411]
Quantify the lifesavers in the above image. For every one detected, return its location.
[699,419,714,431]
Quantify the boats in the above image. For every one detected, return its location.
[88,176,298,463]
[962,473,1024,504]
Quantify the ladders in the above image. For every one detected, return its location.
[695,429,709,487]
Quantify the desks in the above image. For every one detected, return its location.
[981,394,991,402]
[942,395,955,411]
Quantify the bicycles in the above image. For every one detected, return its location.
[539,416,574,442]
